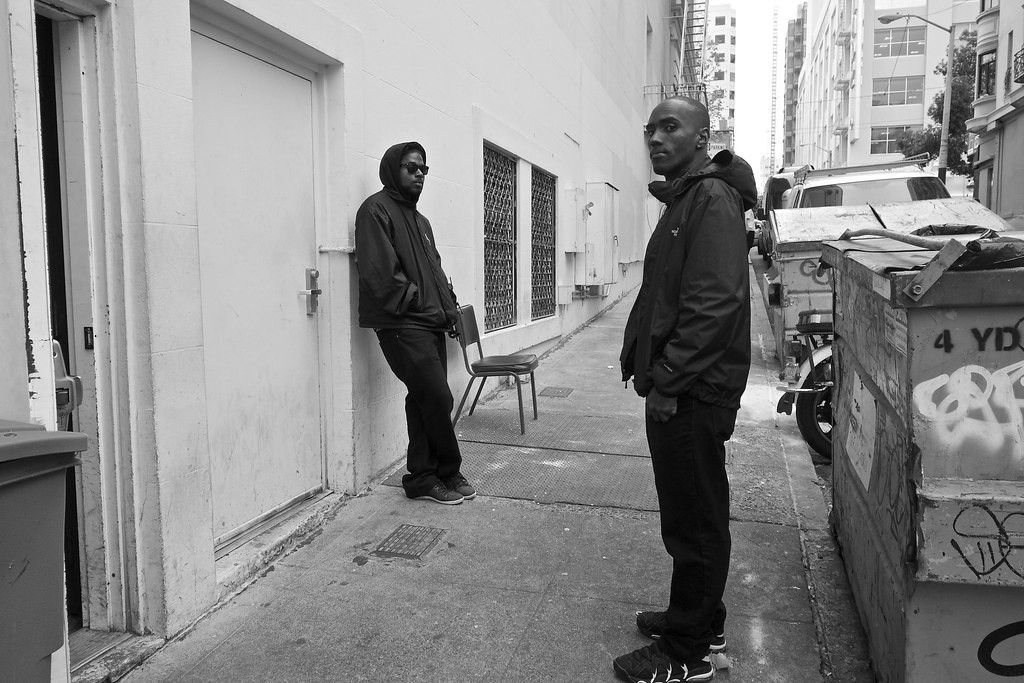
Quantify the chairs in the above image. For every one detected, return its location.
[452,303,539,435]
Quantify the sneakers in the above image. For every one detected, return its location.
[406,477,464,504]
[613,641,714,683]
[436,471,476,499]
[637,611,726,653]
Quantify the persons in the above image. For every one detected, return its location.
[612,95,758,683]
[355,141,477,504]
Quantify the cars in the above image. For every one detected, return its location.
[754,152,952,246]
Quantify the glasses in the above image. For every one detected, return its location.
[400,162,429,175]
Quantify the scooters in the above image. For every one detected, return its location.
[776,308,834,460]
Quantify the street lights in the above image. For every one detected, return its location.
[800,143,832,168]
[877,12,955,185]
